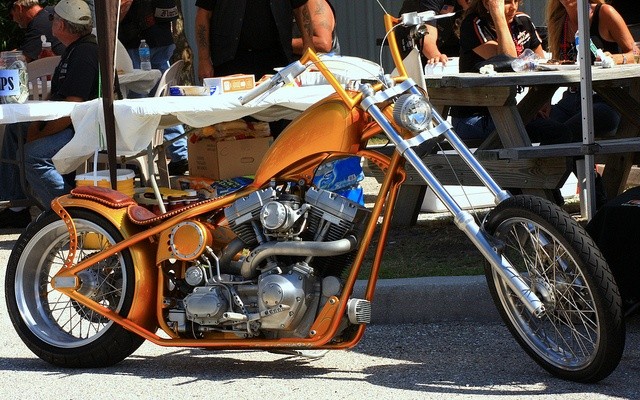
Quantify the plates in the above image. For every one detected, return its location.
[537,63,579,71]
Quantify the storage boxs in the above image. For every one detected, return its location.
[187,136,275,182]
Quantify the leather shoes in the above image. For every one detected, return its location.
[0,207,33,229]
[596,173,608,209]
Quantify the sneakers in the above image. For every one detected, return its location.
[167,160,188,176]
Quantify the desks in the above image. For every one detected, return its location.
[423,62,636,145]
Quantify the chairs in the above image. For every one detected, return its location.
[1,55,63,166]
[87,59,185,192]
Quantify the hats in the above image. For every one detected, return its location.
[54,1,93,25]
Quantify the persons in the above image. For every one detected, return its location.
[547,0,639,210]
[397,0,473,66]
[116,0,189,174]
[7,0,52,62]
[0,1,99,228]
[293,1,341,53]
[195,0,316,139]
[446,0,544,141]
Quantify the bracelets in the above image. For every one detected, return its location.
[624,53,627,64]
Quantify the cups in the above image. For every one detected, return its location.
[511,48,535,72]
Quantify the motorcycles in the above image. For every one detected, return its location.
[5,11,625,384]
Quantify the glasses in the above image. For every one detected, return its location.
[47,13,58,21]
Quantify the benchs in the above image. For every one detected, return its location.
[366,136,639,229]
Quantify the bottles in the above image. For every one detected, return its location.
[0,50,29,104]
[574,30,580,66]
[139,40,151,71]
[38,35,55,81]
